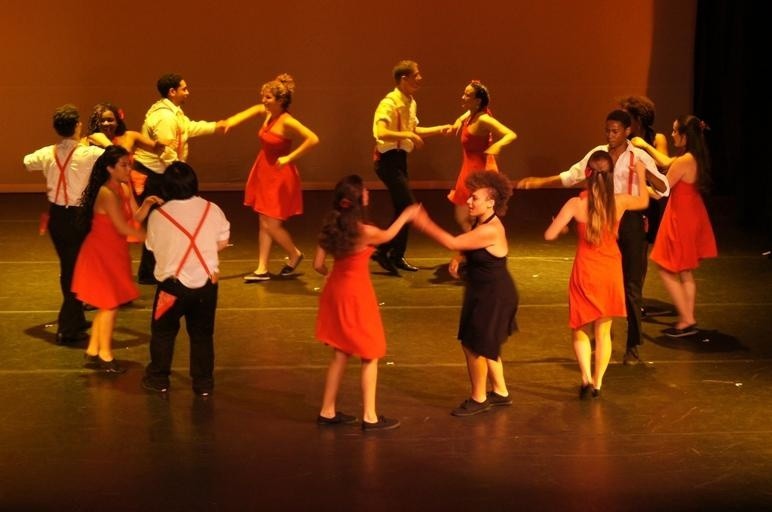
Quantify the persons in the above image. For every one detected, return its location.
[411,169,520,418]
[131,75,227,285]
[312,173,419,432]
[225,72,320,280]
[446,82,517,234]
[617,97,669,315]
[82,103,165,310]
[544,150,649,399]
[516,110,670,365]
[631,114,719,338]
[144,159,232,398]
[24,104,114,344]
[70,145,164,377]
[370,60,451,275]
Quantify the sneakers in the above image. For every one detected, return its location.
[243,272,270,280]
[362,415,401,432]
[592,389,601,398]
[84,303,97,311]
[141,375,168,393]
[451,398,492,416]
[316,411,358,426]
[622,352,640,365]
[487,391,513,406]
[84,353,99,363]
[641,307,647,319]
[579,383,594,401]
[99,357,125,374]
[195,390,209,400]
[139,276,156,285]
[280,252,305,275]
[662,326,698,337]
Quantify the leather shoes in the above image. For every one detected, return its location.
[75,320,92,329]
[398,257,419,271]
[371,251,399,275]
[56,330,89,344]
